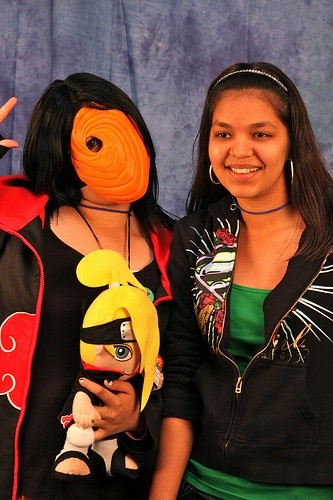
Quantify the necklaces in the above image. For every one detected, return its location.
[235,198,294,215]
[73,203,133,270]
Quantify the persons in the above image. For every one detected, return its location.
[148,62,333,500]
[0,71,176,500]
